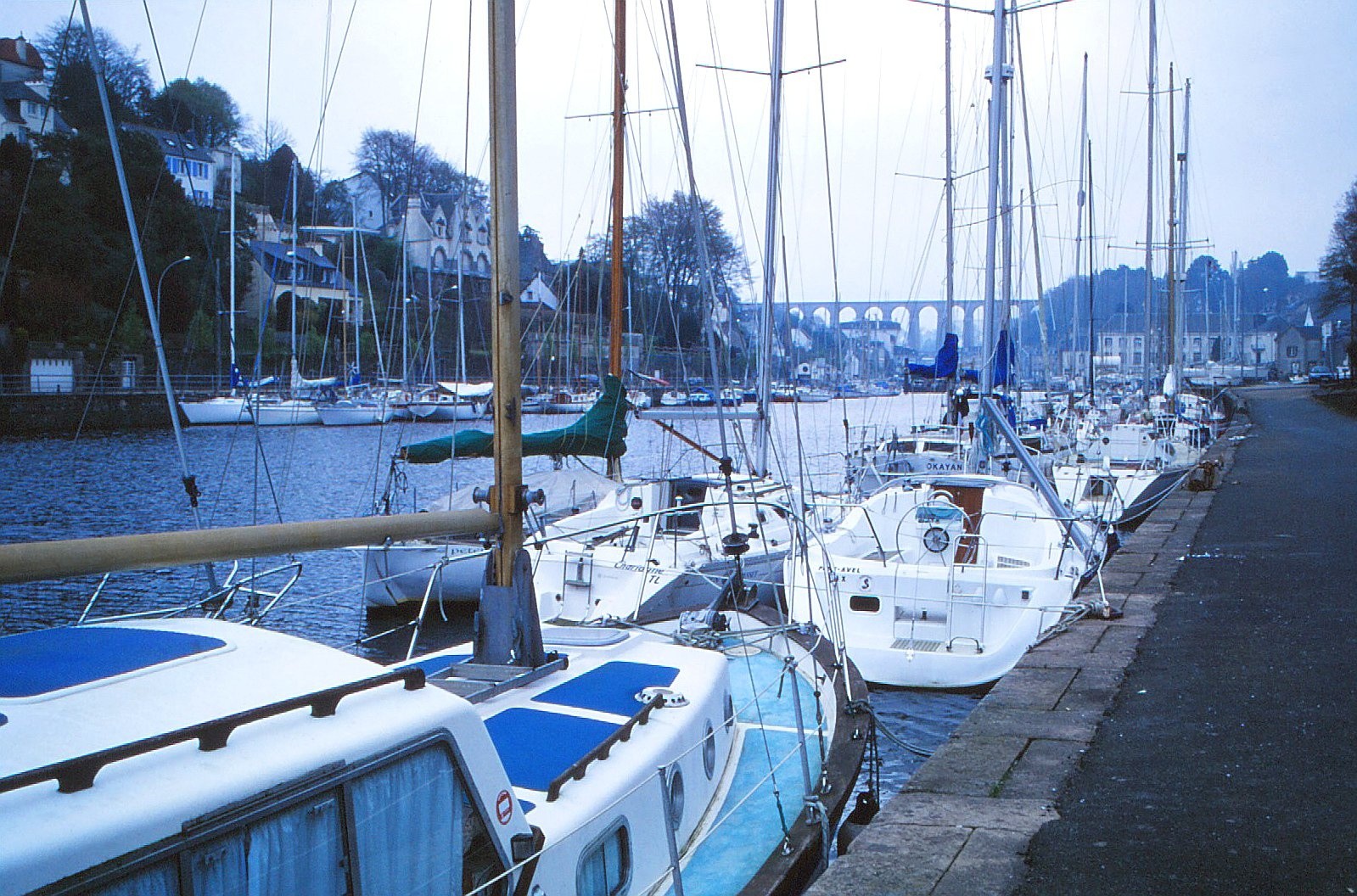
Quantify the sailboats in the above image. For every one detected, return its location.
[176,150,902,433]
[0,0,870,896]
[340,0,1270,693]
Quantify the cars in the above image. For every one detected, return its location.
[1309,366,1333,384]
